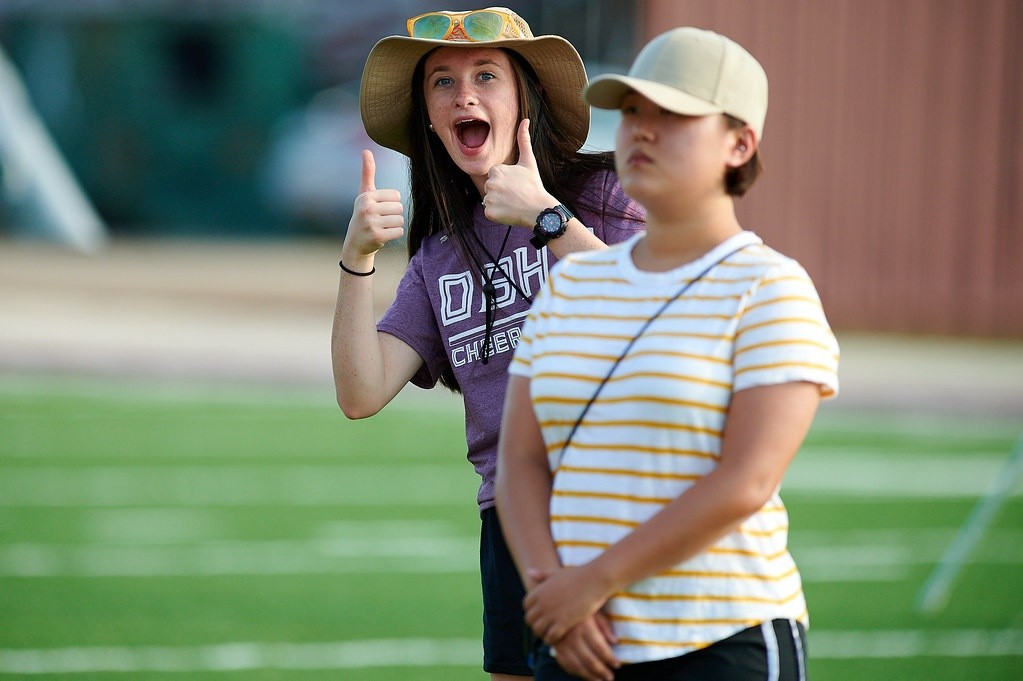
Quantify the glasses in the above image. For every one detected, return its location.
[406,12,525,42]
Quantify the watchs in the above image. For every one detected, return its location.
[529,204,574,250]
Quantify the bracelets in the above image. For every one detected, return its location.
[339,260,375,276]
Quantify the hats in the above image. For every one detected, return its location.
[359,6,592,159]
[580,27,769,142]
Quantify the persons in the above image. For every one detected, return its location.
[332,8,650,680]
[495,28,840,680]
